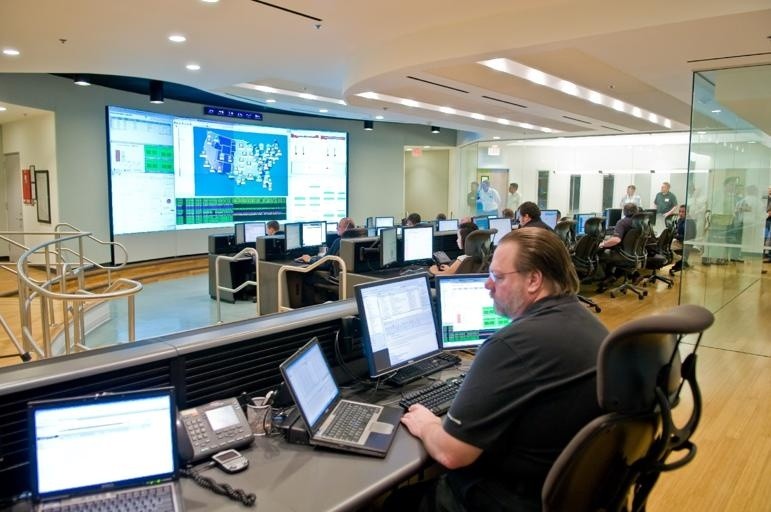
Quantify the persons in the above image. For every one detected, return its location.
[381,226,612,511]
[705,174,771,266]
[402,180,696,295]
[686,186,708,246]
[300,217,356,307]
[245,221,285,299]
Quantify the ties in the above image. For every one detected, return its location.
[354,275,441,378]
[436,274,513,351]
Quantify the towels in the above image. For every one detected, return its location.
[701,213,736,265]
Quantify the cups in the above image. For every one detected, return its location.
[489,269,530,282]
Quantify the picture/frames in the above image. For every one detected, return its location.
[0,349,490,512]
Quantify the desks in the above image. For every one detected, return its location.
[0,349,490,512]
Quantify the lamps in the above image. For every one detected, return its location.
[430,125,440,133]
[149,86,165,104]
[543,302,715,512]
[364,120,374,131]
[71,74,91,87]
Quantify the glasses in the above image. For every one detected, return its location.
[149,86,165,104]
[71,74,91,87]
[430,125,440,133]
[364,120,374,131]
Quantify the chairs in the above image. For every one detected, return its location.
[543,302,715,512]
[671,260,686,271]
[552,212,695,300]
[602,274,617,285]
[242,222,498,306]
[633,275,644,285]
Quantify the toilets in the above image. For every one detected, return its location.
[175,397,255,462]
[433,251,452,264]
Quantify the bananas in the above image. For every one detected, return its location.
[388,353,461,383]
[399,373,468,417]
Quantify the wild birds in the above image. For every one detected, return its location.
[279,336,403,457]
[24,386,181,512]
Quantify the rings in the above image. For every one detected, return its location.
[241,384,279,406]
